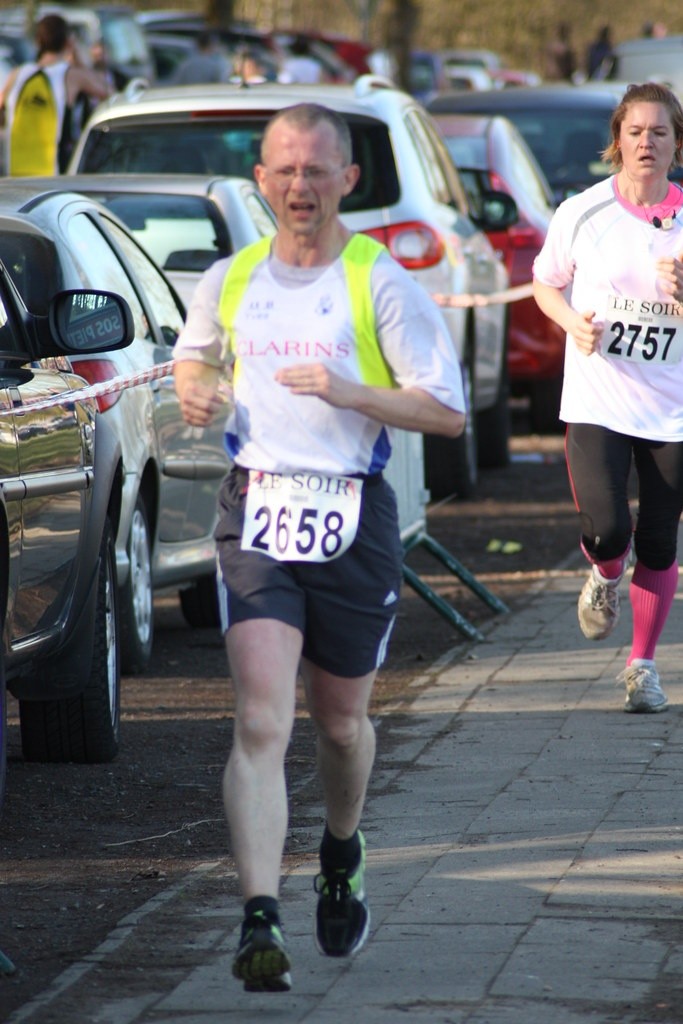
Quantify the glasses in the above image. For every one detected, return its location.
[261,164,348,185]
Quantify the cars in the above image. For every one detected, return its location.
[429,115,582,435]
[2,174,278,314]
[1,12,683,244]
[0,188,237,764]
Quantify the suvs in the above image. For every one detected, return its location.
[65,73,514,502]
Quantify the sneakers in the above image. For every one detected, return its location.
[578,549,634,640]
[313,824,370,960]
[616,658,668,713]
[231,909,293,992]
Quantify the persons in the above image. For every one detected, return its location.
[532,83,683,713]
[170,102,466,994]
[79,43,126,127]
[586,25,616,80]
[171,33,225,85]
[233,52,266,84]
[546,23,573,81]
[0,15,111,176]
